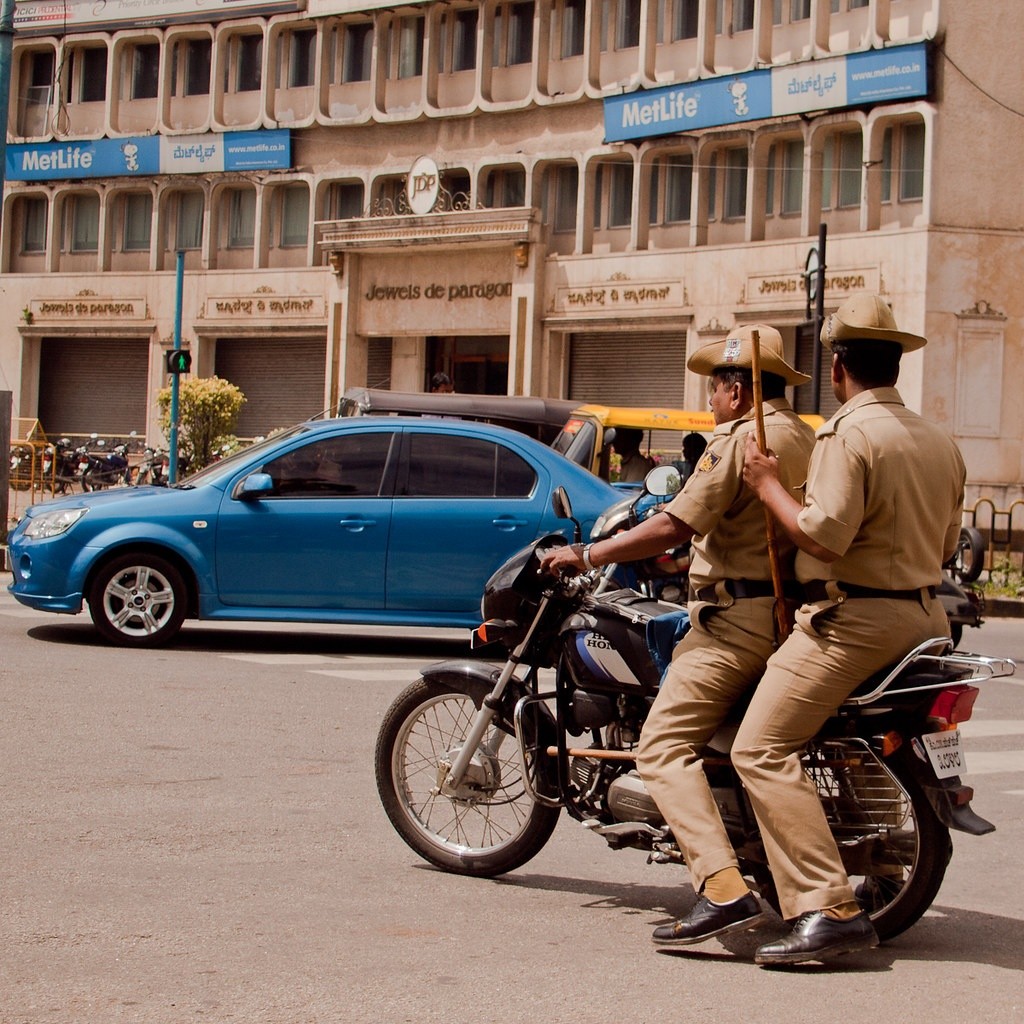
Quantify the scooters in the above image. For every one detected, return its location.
[937,527,985,649]
[9,431,169,493]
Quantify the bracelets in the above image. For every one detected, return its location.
[583,543,595,570]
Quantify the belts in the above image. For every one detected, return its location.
[798,580,937,604]
[698,579,803,604]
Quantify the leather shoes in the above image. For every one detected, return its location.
[652,889,766,944]
[755,909,880,963]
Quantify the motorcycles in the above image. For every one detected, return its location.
[374,465,1017,942]
[340,388,827,484]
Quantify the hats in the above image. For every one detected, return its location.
[820,294,928,353]
[686,322,813,387]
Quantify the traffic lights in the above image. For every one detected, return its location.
[166,349,192,374]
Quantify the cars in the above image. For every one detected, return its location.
[5,416,677,649]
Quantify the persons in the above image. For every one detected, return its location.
[540,323,817,945]
[612,427,649,482]
[730,293,967,964]
[682,432,707,487]
[422,372,462,420]
[287,436,373,496]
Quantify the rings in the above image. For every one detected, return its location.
[776,455,779,458]
